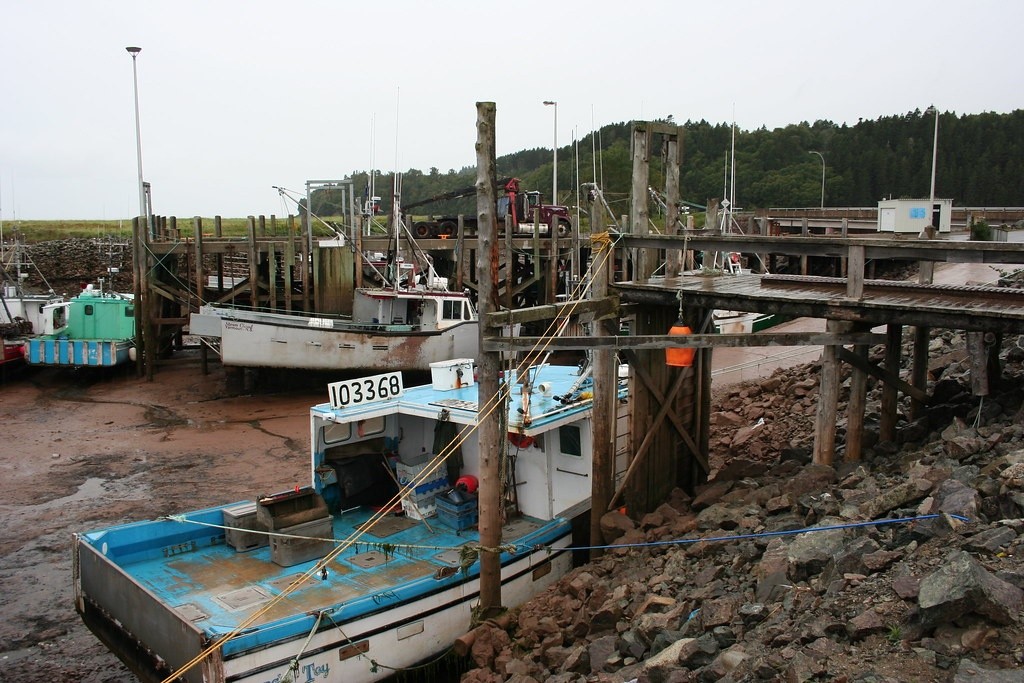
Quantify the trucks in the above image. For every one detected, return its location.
[410,190,574,239]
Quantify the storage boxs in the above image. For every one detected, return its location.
[223,487,335,568]
[429,358,474,391]
[395,453,478,531]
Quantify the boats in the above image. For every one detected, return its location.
[187,285,506,370]
[0,287,64,365]
[64,358,638,683]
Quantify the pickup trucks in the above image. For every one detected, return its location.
[29,288,145,372]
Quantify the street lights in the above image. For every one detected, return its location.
[125,46,146,226]
[540,97,558,210]
[926,106,938,208]
[808,149,828,208]
